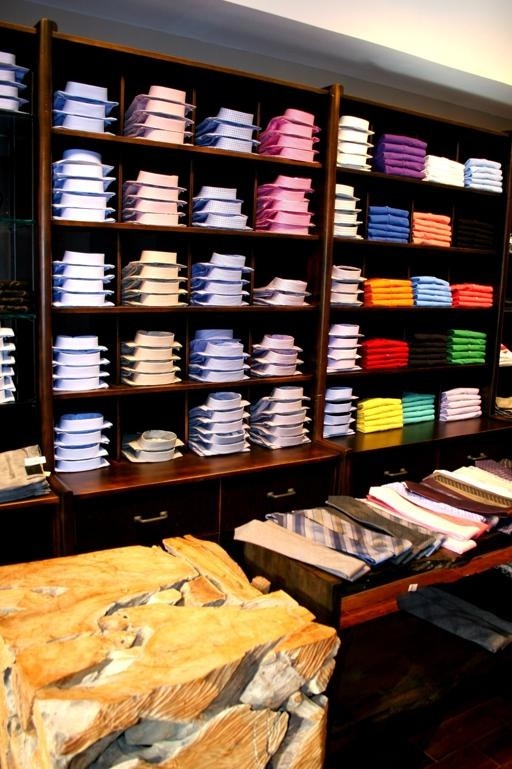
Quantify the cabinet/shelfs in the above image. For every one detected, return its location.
[0,17,512,469]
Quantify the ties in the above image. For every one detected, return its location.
[233,459,512,583]
[396,586,512,653]
